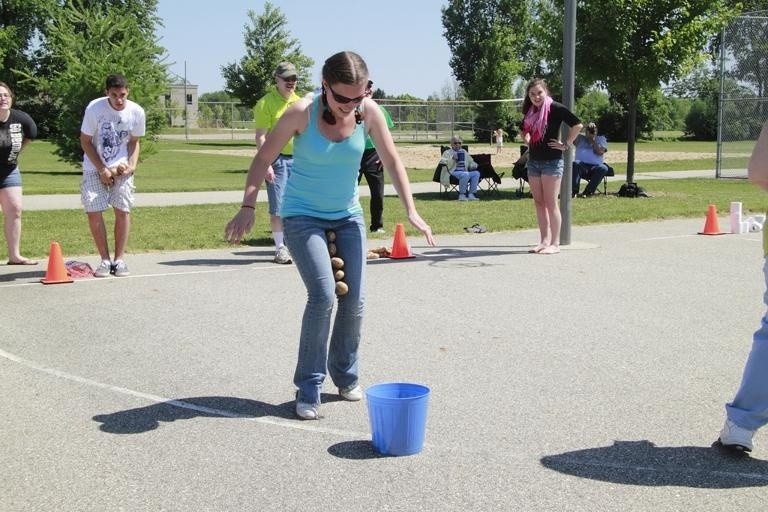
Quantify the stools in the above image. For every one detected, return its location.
[578,174,608,195]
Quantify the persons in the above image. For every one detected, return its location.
[716,120,767,452]
[519,79,583,254]
[254,61,302,264]
[439,135,480,201]
[572,122,608,198]
[515,123,530,157]
[356,79,394,234]
[79,74,146,278]
[0,81,39,265]
[224,51,435,420]
[493,128,504,154]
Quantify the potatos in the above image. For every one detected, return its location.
[367,246,387,259]
[327,230,349,296]
[110,163,126,177]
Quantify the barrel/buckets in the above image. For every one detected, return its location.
[364,382,431,457]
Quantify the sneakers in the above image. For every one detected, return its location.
[275,246,293,264]
[718,420,757,453]
[295,389,321,421]
[94,260,130,277]
[339,383,363,400]
[458,193,480,202]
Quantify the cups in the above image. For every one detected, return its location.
[730,201,750,234]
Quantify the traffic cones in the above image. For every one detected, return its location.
[387,223,417,259]
[40,240,77,285]
[698,204,731,236]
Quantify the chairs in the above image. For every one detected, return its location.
[469,153,504,198]
[435,145,468,199]
[512,144,532,198]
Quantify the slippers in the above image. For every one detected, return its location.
[463,222,488,234]
[7,258,39,266]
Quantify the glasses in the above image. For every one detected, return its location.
[327,82,366,105]
[280,76,297,82]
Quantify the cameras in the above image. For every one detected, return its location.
[589,128,595,134]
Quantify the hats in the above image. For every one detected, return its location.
[276,61,298,81]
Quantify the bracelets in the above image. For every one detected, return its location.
[241,205,255,210]
[99,167,108,175]
